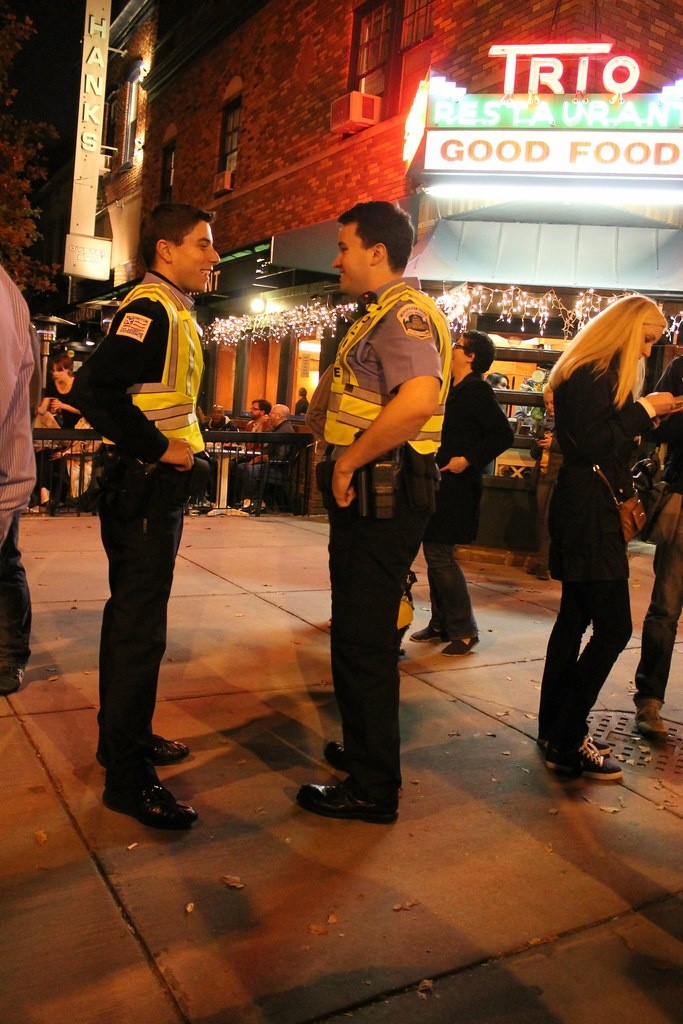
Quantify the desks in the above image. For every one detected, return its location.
[207,449,262,517]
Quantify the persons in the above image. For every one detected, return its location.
[527,383,563,581]
[485,373,510,475]
[72,203,220,828]
[37,355,82,428]
[29,417,103,513]
[536,295,683,780]
[0,264,36,692]
[295,388,309,415]
[195,404,238,431]
[227,398,294,512]
[296,201,452,823]
[409,331,515,658]
[633,355,683,742]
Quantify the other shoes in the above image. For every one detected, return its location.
[442,637,479,656]
[633,696,667,740]
[536,564,549,579]
[410,626,449,641]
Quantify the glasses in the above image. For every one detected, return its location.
[452,343,465,350]
[251,407,261,410]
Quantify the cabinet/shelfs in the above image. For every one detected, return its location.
[477,311,683,449]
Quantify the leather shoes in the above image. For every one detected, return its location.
[103,781,198,829]
[297,784,398,823]
[97,734,189,766]
[325,741,350,771]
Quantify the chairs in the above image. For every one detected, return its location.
[255,444,312,517]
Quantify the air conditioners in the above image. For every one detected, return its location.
[212,171,235,195]
[329,91,382,135]
[99,155,112,177]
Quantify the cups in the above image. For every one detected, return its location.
[521,425,530,436]
[49,398,58,413]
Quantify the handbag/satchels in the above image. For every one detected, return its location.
[618,488,646,544]
[631,451,683,547]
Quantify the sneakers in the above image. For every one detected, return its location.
[0,666,25,693]
[538,733,610,756]
[546,738,624,779]
